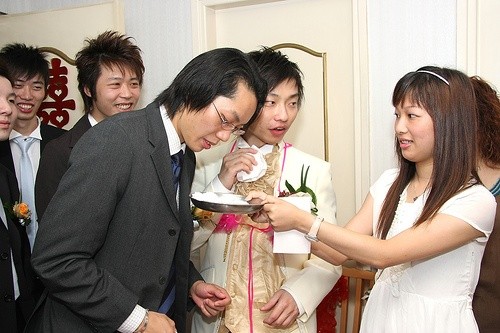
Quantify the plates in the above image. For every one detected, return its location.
[188,191,265,213]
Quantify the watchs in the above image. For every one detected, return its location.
[305,216,324,242]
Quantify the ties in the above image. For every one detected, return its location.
[171,152,181,198]
[10,137,38,254]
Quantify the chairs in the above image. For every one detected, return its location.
[336,266,376,333]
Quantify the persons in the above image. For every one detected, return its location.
[0,65,33,333]
[34,30,145,228]
[188,46,343,333]
[0,42,68,256]
[245,66,497,333]
[31,48,268,333]
[470,76,500,333]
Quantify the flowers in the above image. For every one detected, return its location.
[277,165,320,217]
[6,197,32,227]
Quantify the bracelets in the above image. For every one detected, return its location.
[133,308,149,333]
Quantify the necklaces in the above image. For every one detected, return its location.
[413,179,432,201]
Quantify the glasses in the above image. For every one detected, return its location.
[211,100,246,138]
